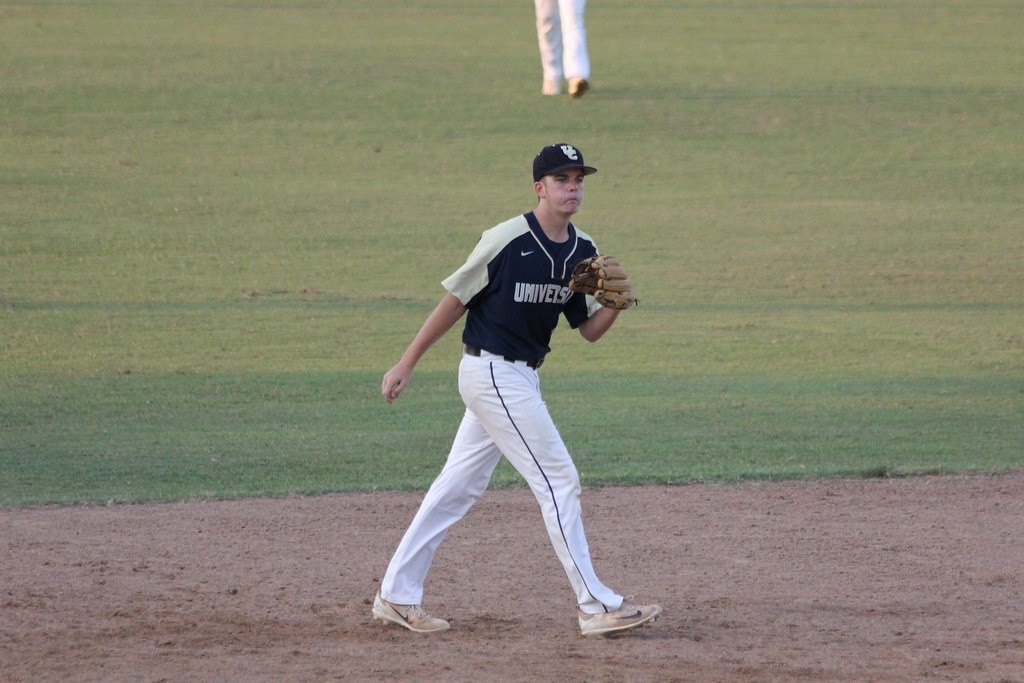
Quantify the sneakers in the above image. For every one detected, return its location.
[576,595,664,637]
[372,587,450,632]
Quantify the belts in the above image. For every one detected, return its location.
[463,344,544,370]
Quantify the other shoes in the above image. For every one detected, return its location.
[542,78,562,96]
[567,77,588,98]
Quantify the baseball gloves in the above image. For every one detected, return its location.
[568,253,633,310]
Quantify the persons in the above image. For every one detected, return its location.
[372,142,663,637]
[534,0,589,96]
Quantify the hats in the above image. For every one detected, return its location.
[533,143,597,181]
